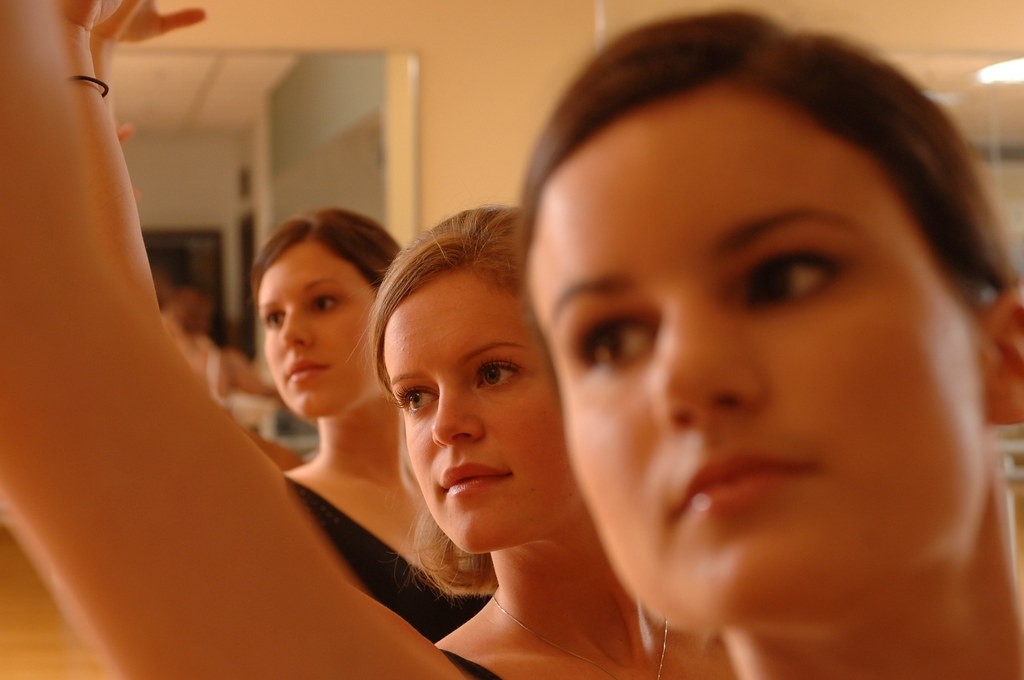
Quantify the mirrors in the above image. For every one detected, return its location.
[110,45,420,452]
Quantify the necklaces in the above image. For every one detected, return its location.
[486,596,669,678]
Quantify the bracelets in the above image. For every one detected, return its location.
[65,74,109,99]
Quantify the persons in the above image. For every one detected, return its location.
[219,405,305,473]
[60,0,736,679]
[1,2,1024,680]
[89,0,500,646]
[147,240,280,424]
